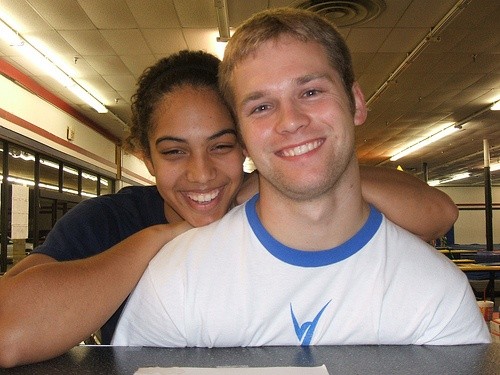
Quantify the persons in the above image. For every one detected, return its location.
[0,50,463,370]
[108,6,494,347]
